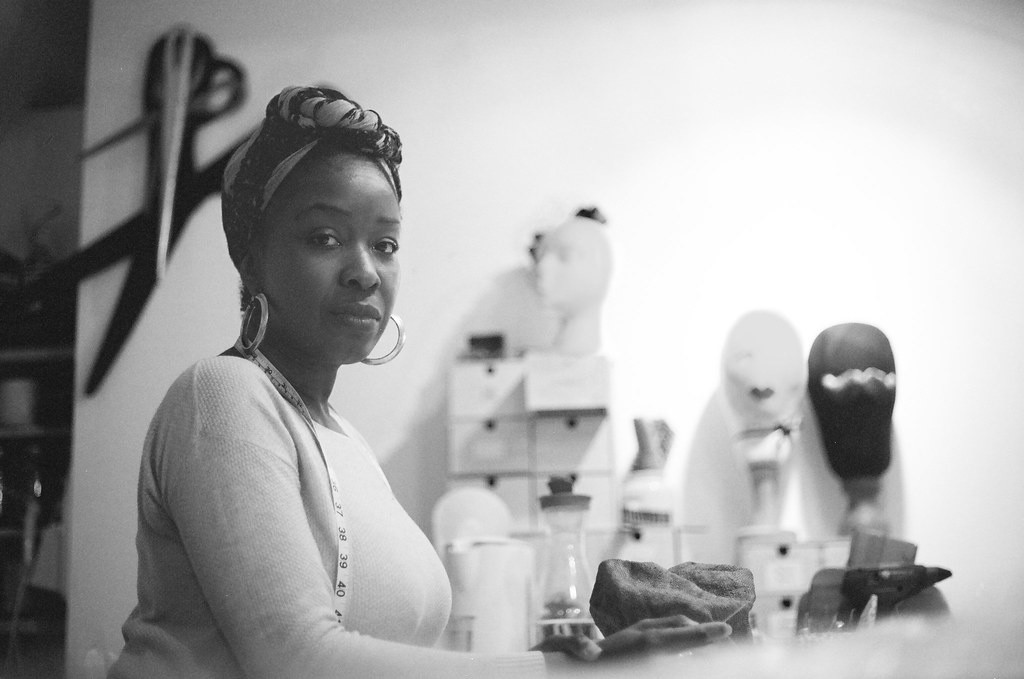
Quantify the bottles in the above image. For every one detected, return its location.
[538,474,597,642]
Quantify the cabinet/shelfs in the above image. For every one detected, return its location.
[450,354,625,596]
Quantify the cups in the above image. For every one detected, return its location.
[2,379,37,426]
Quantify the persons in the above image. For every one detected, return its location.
[108,83,733,679]
